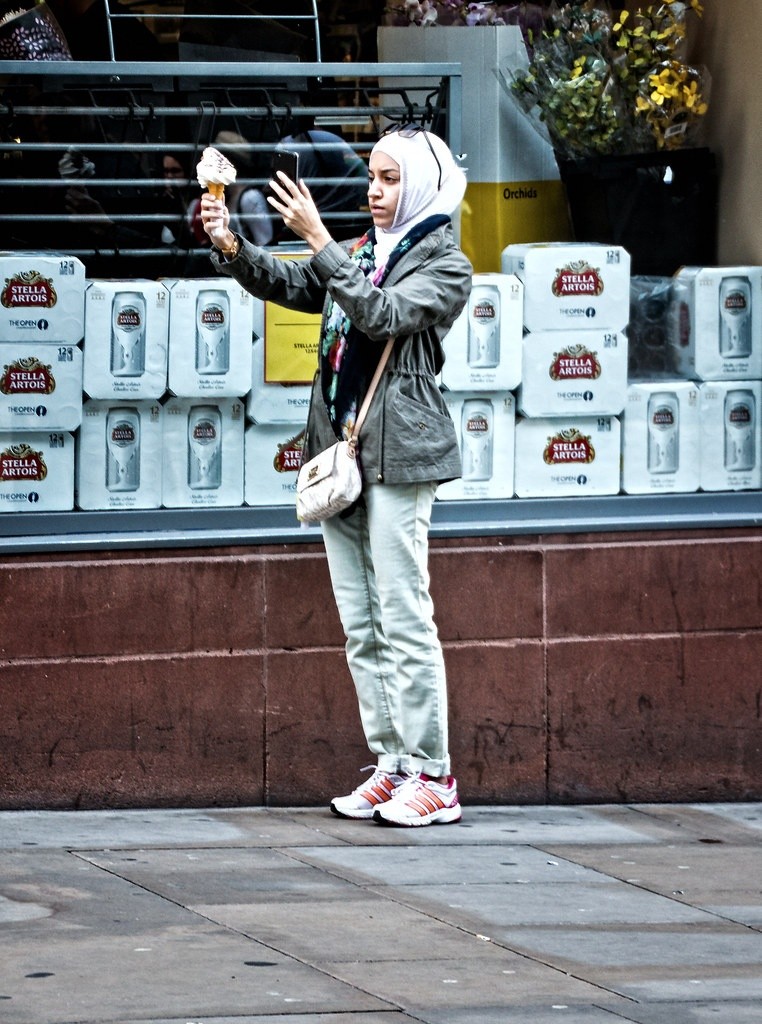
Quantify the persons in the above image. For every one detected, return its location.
[147,146,210,278]
[186,130,277,278]
[198,129,473,829]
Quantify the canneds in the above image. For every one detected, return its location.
[102,275,757,493]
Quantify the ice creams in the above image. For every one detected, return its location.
[196,147,237,238]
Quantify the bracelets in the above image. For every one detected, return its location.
[221,237,239,258]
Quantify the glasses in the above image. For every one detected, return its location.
[380,122,442,192]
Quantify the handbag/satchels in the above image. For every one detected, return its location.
[295,441,363,523]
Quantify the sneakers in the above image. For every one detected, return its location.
[328,771,462,825]
